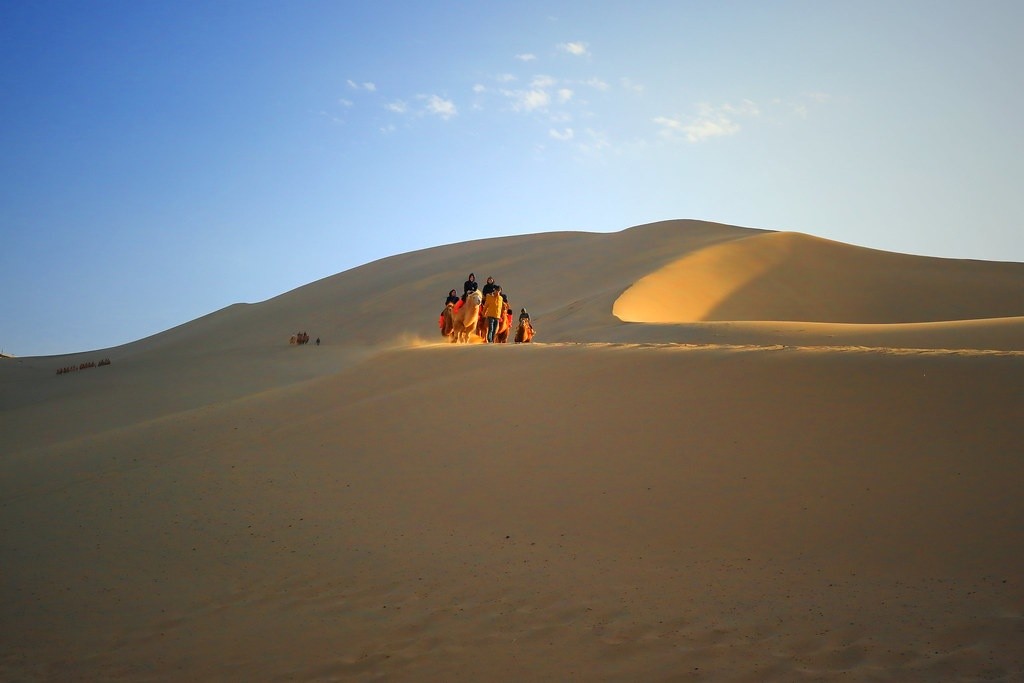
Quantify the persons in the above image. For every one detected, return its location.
[316,337,320,345]
[439,289,459,328]
[479,276,512,328]
[56,358,110,373]
[481,286,503,343]
[304,331,307,337]
[297,331,302,337]
[515,308,536,336]
[453,273,478,314]
[291,333,295,340]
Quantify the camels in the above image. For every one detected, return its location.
[514,318,533,344]
[442,290,510,344]
